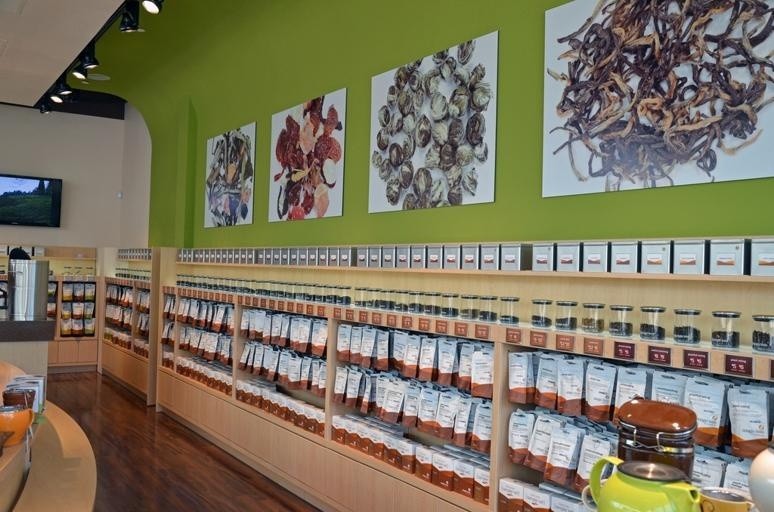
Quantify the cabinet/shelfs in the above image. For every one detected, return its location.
[0,238,99,372]
[96,236,774,512]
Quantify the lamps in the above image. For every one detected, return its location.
[33,0,164,113]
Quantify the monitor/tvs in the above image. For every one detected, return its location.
[0,173,63,228]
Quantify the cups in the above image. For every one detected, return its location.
[699,487,756,512]
[0,405,35,448]
[747,441,773,511]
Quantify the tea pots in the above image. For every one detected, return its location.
[588,456,702,512]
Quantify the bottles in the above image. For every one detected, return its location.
[752,314,773,353]
[582,301,605,335]
[529,298,554,329]
[111,265,520,327]
[673,308,702,345]
[556,301,578,329]
[62,266,92,276]
[639,305,667,340]
[711,311,741,350]
[616,396,698,482]
[0,265,4,274]
[608,304,635,337]
